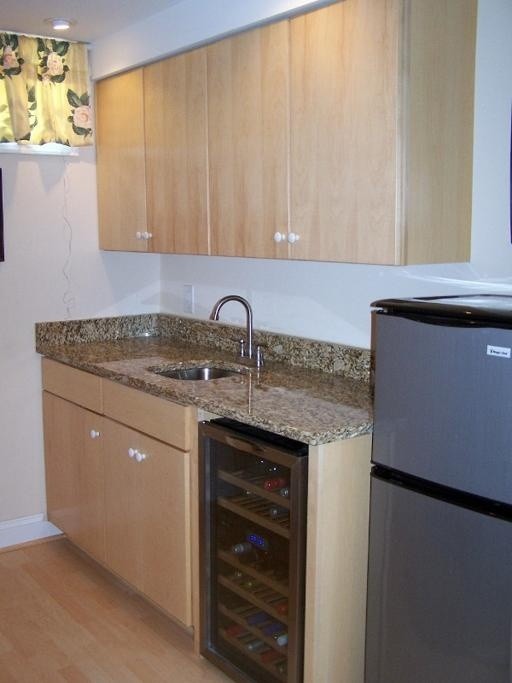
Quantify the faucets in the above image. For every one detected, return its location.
[209,295,253,359]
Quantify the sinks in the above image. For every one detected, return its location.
[143,359,250,381]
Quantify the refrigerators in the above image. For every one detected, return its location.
[360,291,512,682]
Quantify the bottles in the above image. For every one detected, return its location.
[218,454,292,670]
